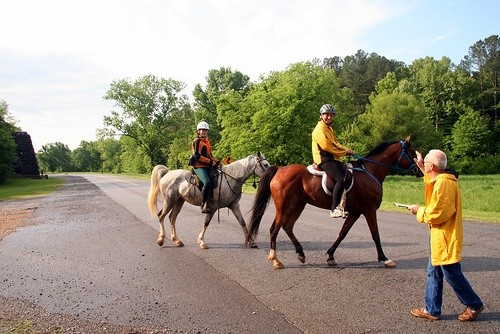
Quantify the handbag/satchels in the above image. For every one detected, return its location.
[188,156,196,166]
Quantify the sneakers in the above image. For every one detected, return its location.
[411,307,439,320]
[458,305,485,321]
[329,208,349,218]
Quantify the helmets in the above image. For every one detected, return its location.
[320,104,335,113]
[196,121,209,130]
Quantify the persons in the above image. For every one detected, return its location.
[407,150,485,322]
[311,104,356,219]
[188,121,219,214]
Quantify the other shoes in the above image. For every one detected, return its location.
[201,204,210,213]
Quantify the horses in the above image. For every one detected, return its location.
[244,135,424,269]
[148,151,270,249]
